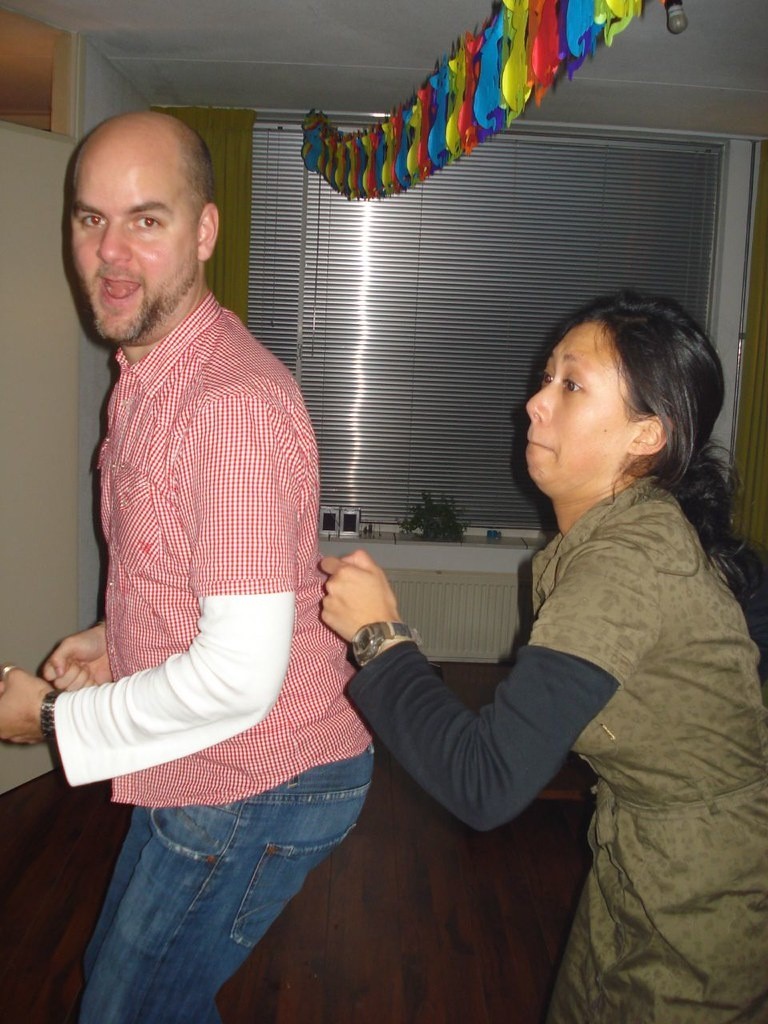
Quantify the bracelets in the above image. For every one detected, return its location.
[40,689,65,739]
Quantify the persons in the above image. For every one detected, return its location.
[0,109,374,1024]
[320,288,768,1024]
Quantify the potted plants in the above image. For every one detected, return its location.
[397,497,472,544]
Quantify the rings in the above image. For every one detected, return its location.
[2,664,16,680]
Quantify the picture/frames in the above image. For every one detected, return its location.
[318,506,363,536]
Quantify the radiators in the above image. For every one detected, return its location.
[384,569,532,664]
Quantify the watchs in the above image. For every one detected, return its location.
[352,622,423,667]
